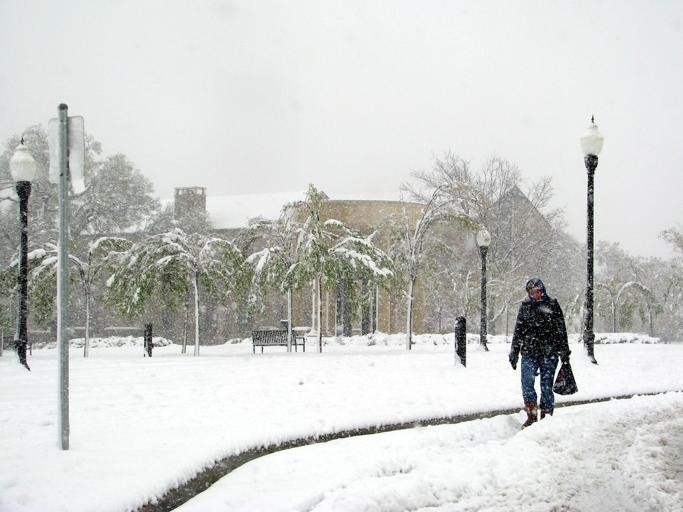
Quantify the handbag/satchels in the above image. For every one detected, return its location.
[552,362,579,396]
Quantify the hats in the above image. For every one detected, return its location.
[525,278,546,292]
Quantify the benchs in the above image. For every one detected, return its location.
[252,320,309,352]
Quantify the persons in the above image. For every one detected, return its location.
[508,276,570,432]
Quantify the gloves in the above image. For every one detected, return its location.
[509,351,519,371]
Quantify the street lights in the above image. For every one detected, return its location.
[7,136,37,371]
[579,113,605,365]
[476,224,492,353]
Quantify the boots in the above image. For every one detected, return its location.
[521,401,538,430]
[540,404,553,419]
